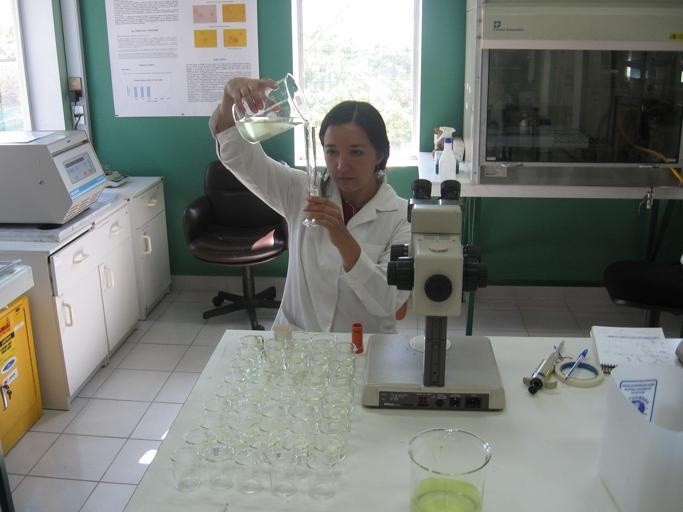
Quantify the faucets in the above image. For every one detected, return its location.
[639,182,654,212]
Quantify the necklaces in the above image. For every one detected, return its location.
[344,197,357,217]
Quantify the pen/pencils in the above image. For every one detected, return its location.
[564,349,588,380]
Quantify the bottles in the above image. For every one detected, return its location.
[350,321,364,353]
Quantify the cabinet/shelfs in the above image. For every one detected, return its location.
[49,204,138,414]
[129,180,172,321]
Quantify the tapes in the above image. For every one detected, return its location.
[554,360,604,388]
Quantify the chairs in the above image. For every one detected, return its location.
[184,155,290,331]
[606,261,683,335]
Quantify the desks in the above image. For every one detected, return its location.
[415,151,682,337]
[123,329,683,512]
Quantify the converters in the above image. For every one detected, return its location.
[69,91,79,103]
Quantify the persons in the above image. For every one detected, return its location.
[209,76,413,334]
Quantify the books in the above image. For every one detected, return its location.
[591,321,680,374]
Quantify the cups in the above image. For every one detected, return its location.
[406,427,494,512]
[232,73,311,145]
[171,334,357,498]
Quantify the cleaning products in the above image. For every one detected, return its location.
[434,126,457,181]
